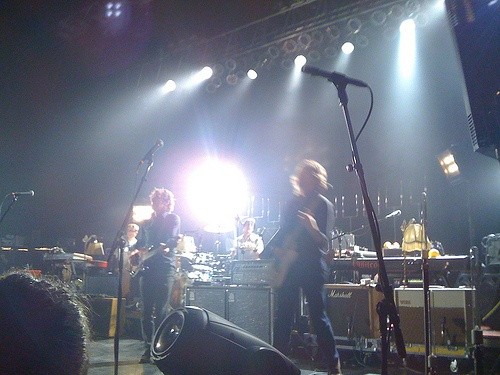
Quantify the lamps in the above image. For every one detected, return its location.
[246,59,268,80]
[150,305,301,375]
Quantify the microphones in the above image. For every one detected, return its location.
[301,65,368,87]
[440,316,446,335]
[7,191,35,196]
[386,210,401,220]
[140,139,164,164]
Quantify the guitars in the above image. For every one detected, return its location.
[125,234,185,277]
[267,177,319,288]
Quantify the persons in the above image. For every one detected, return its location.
[127,224,139,247]
[136,188,180,364]
[272,160,342,375]
[231,218,264,259]
[0,271,90,375]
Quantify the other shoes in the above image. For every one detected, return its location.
[328,366,344,375]
[139,352,150,363]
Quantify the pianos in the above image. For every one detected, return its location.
[332,248,471,347]
[43,252,93,286]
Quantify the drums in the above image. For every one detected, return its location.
[189,251,214,271]
[212,254,233,273]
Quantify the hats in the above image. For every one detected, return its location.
[242,218,255,224]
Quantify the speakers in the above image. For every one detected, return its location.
[445,0,500,159]
[85,297,126,337]
[185,284,277,347]
[394,286,477,356]
[323,284,383,341]
